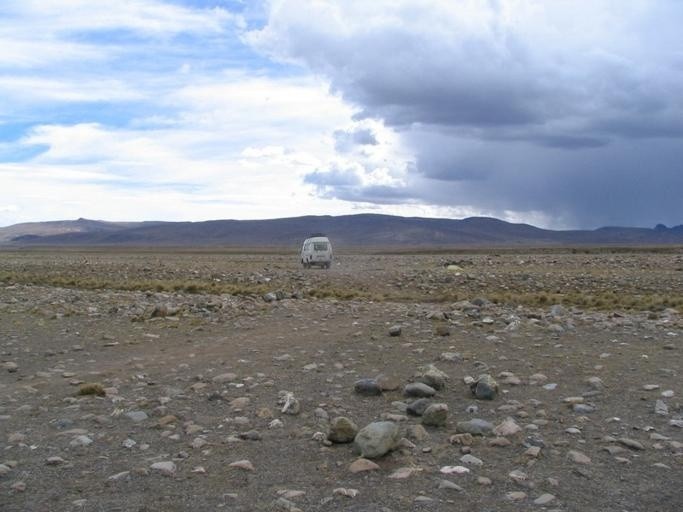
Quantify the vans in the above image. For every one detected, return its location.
[301,236,333,269]
